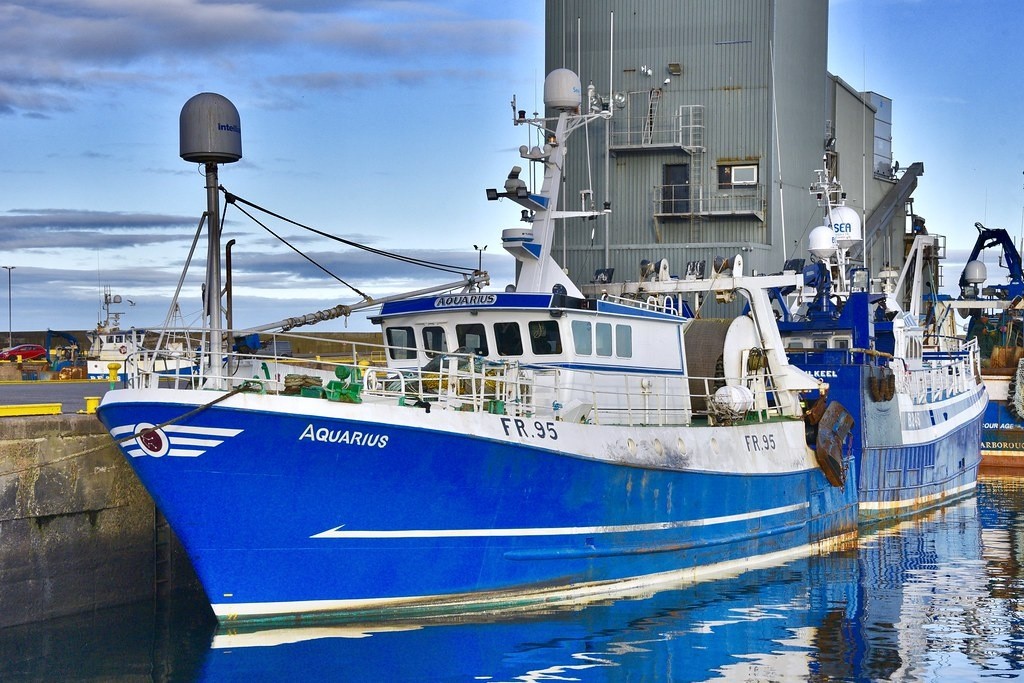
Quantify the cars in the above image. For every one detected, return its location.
[0,344,46,361]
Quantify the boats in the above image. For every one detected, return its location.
[95,68,988,620]
[941,221,1024,468]
[22,294,237,382]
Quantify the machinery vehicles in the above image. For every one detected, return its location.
[46,329,87,371]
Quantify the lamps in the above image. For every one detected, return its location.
[516,187,528,199]
[590,93,603,107]
[668,63,681,74]
[613,92,626,108]
[486,189,497,200]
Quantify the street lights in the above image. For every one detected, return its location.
[2,267,17,347]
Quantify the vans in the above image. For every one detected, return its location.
[257,341,293,357]
